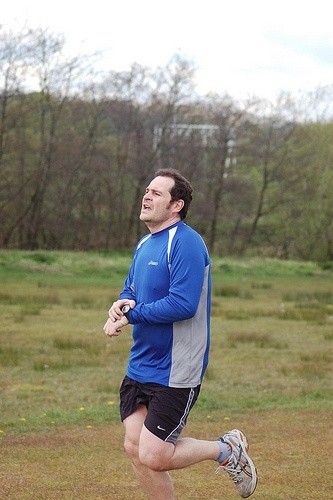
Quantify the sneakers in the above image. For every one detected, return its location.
[215,428,259,499]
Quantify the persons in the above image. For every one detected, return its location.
[103,168,258,500]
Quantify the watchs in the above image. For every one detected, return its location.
[122,305,130,319]
